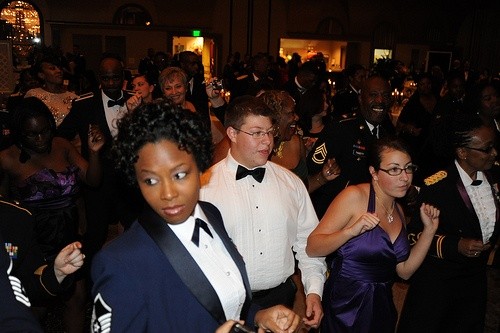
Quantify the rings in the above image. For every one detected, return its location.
[475,251,480,256]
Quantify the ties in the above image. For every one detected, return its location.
[187,83,191,96]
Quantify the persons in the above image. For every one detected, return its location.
[396,113,500,333]
[0,46,500,333]
[90,99,301,333]
[306,133,440,333]
[194,98,328,332]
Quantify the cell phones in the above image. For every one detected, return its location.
[229,322,251,333]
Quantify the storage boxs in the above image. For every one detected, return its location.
[412,47,452,72]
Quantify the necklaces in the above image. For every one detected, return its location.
[273,141,286,158]
[376,195,394,223]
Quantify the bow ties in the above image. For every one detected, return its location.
[191,218,213,247]
[108,98,125,107]
[235,164,266,183]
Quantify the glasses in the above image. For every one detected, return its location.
[378,163,417,176]
[372,128,377,138]
[462,144,491,154]
[232,126,280,139]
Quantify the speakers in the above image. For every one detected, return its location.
[0,40,16,94]
